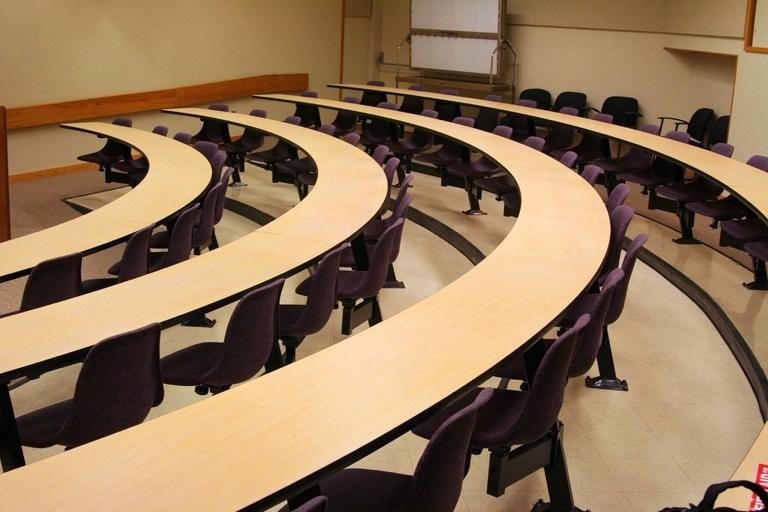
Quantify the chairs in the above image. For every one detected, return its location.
[61,80,768,245]
[1,182,768,512]
